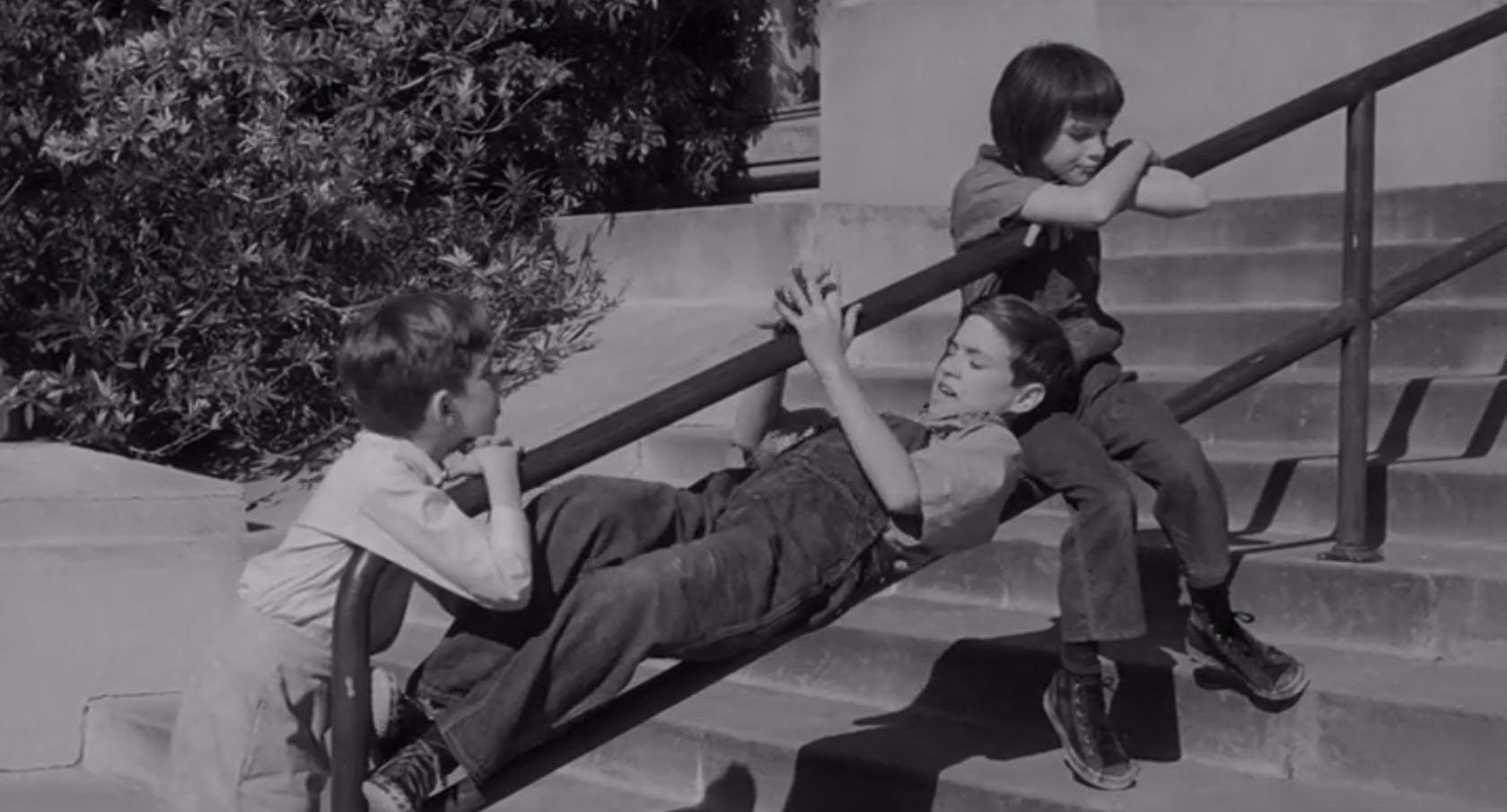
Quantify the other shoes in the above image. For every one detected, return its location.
[362,740,440,812]
[367,666,431,764]
[1184,603,1309,700]
[1042,670,1139,791]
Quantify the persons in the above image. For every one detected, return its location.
[950,42,1309,792]
[361,255,1078,812]
[173,287,535,811]
